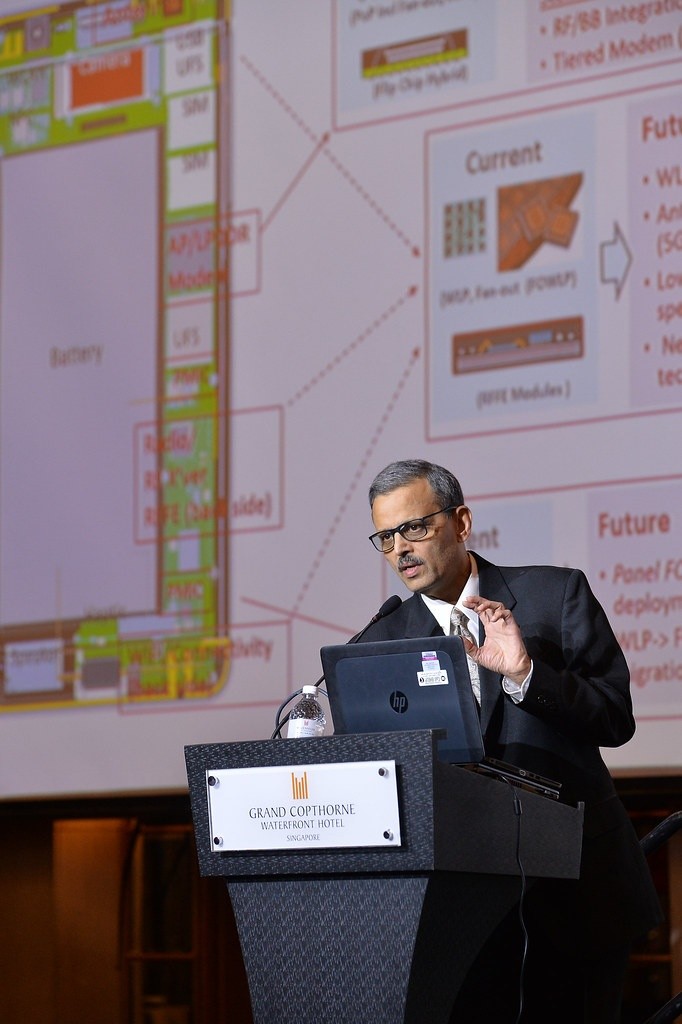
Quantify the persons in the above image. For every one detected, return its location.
[346,460,666,1024]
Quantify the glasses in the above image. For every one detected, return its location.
[368,505,458,553]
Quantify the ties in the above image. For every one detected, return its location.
[450,607,481,709]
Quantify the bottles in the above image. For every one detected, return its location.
[287,685,326,739]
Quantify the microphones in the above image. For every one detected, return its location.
[268,595,402,738]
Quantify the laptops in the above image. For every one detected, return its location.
[321,635,559,801]
